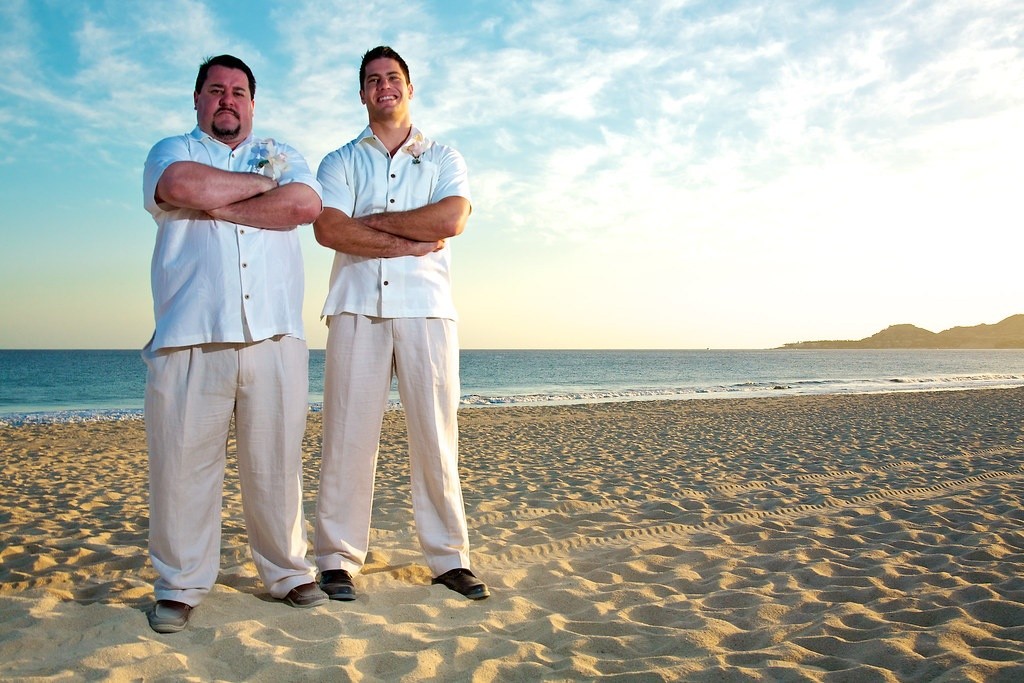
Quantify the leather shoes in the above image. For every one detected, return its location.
[319,569,356,600]
[286,582,330,608]
[431,568,490,599]
[148,600,191,632]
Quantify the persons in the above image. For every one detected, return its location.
[142,54,328,633]
[311,44,490,602]
